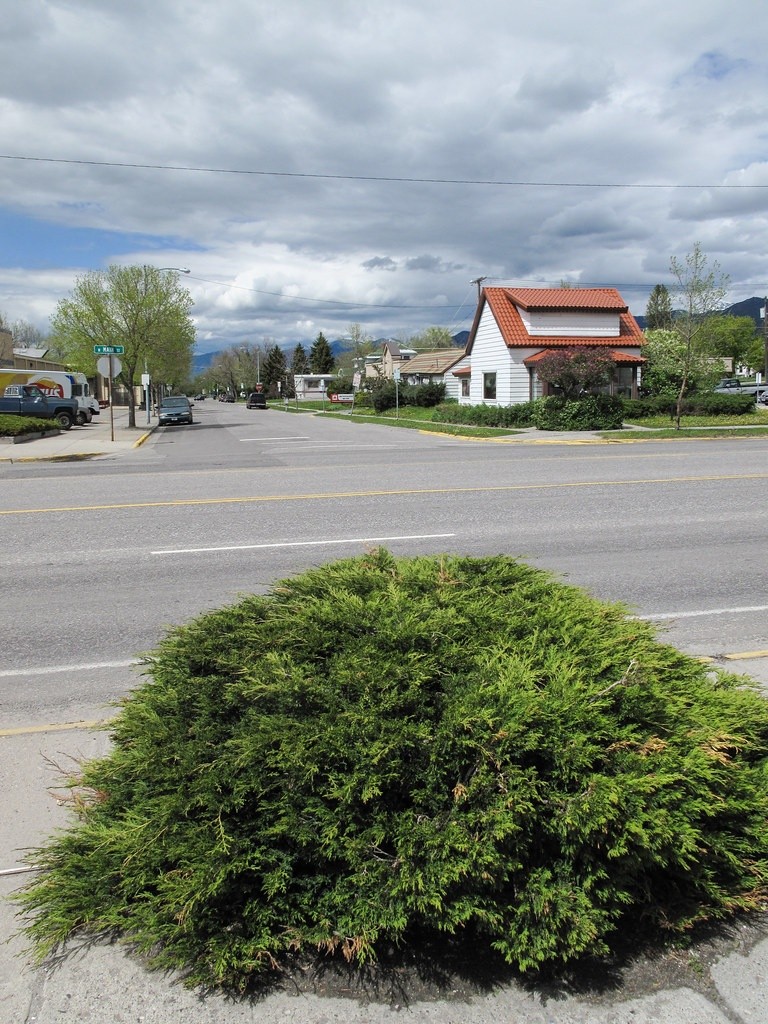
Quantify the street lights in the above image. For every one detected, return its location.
[142,266,191,423]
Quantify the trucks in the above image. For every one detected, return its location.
[0,369,100,426]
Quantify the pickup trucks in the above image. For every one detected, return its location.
[0,384,79,430]
[710,379,768,403]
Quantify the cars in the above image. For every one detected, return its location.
[155,396,194,426]
[757,392,768,406]
[194,395,205,401]
[219,395,235,403]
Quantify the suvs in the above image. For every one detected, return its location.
[246,393,266,409]
